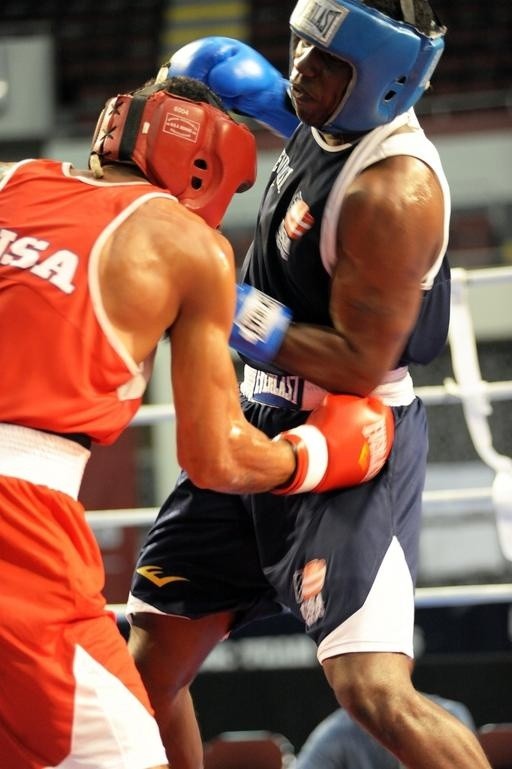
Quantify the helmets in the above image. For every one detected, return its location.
[290,0,447,131]
[92,92,257,224]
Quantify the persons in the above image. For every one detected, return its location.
[1,77,396,769]
[129,3,491,769]
[295,631,471,769]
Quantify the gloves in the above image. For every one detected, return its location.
[272,396,394,497]
[160,36,300,139]
[227,284,291,371]
